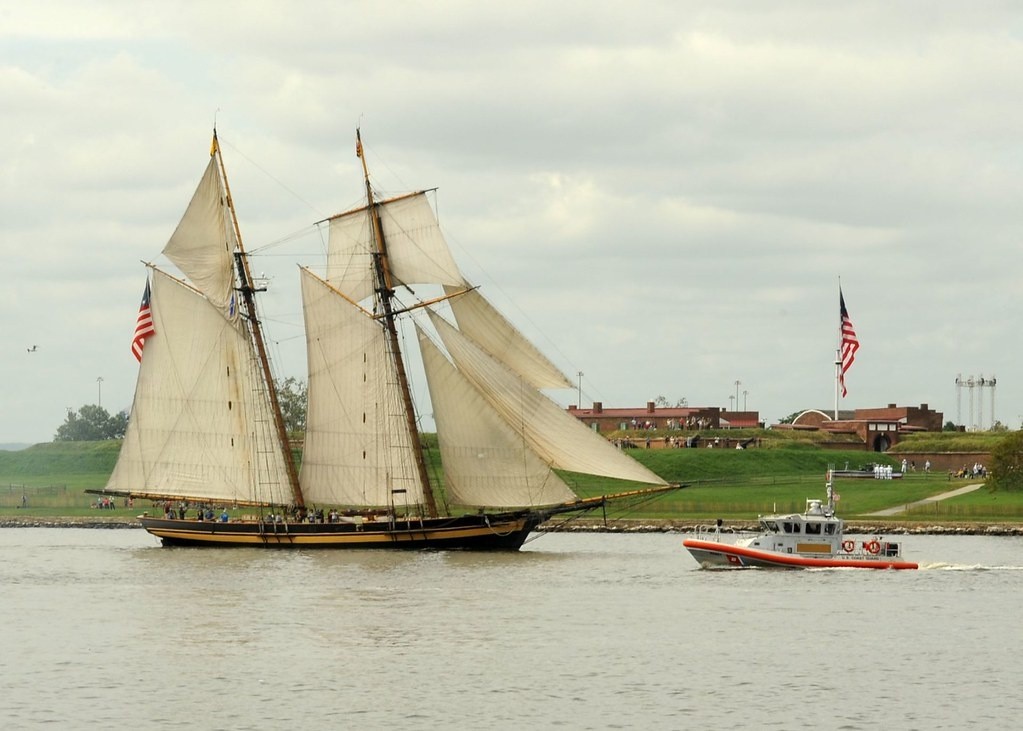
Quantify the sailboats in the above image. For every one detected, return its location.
[82,109,693,553]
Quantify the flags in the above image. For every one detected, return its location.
[130,274,157,363]
[840,287,861,398]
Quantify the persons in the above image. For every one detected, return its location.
[948,462,988,481]
[205,507,215,520]
[328,509,337,523]
[707,442,713,448]
[220,508,228,522]
[665,416,712,430]
[179,506,187,520]
[319,509,324,524]
[630,417,657,430]
[164,501,177,520]
[715,435,719,446]
[901,458,908,473]
[911,460,916,472]
[663,435,691,448]
[21,493,27,508]
[276,511,282,523]
[197,510,203,522]
[925,459,931,473]
[607,435,650,449]
[98,495,115,511]
[124,495,133,512]
[308,509,315,523]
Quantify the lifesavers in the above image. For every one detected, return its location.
[825,471,831,483]
[842,540,855,553]
[868,541,881,554]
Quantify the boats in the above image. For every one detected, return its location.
[683,462,920,571]
